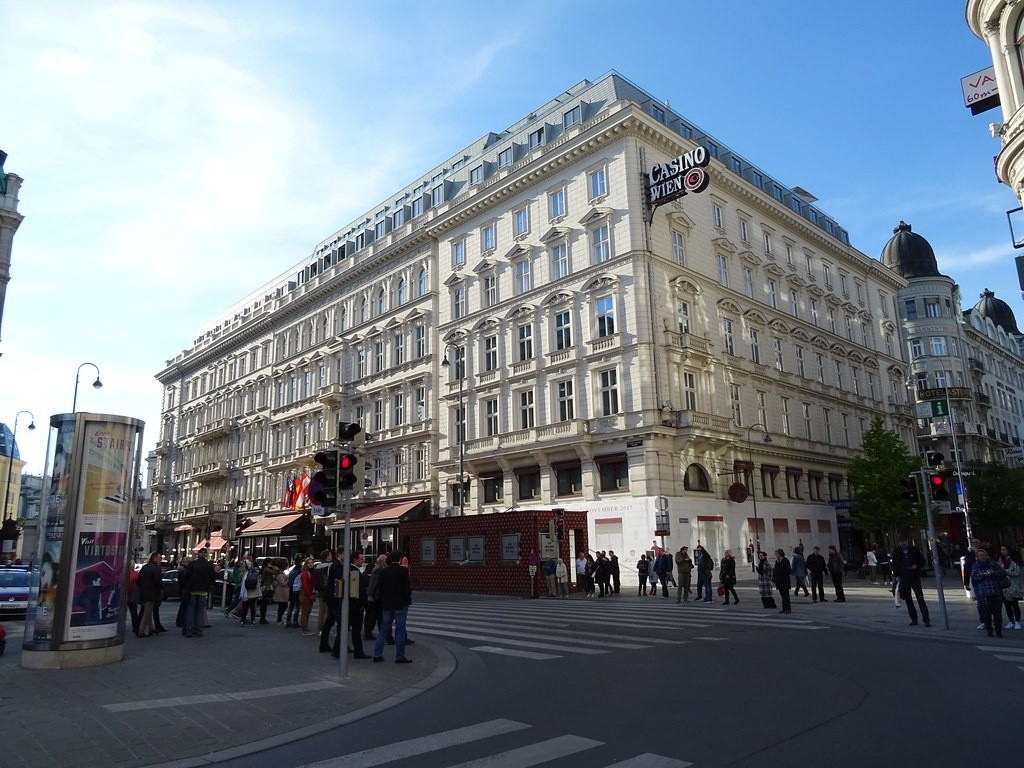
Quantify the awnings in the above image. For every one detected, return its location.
[192,526,240,554]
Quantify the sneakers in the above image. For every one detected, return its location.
[1015,621,1021,629]
[1004,622,1015,628]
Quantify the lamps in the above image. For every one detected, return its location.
[989,122,1001,137]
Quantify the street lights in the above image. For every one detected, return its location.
[748,423,773,551]
[905,354,973,551]
[0,411,36,549]
[440,342,464,516]
[73,362,103,414]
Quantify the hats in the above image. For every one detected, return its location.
[198,548,207,554]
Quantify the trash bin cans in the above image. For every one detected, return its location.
[959,555,977,599]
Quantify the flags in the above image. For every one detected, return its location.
[281,469,316,516]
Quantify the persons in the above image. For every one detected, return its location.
[128,544,415,664]
[805,547,829,602]
[6,553,34,571]
[936,539,1024,636]
[637,545,714,604]
[827,545,847,602]
[719,550,740,605]
[895,537,931,627]
[772,549,792,614]
[755,551,777,608]
[857,546,894,586]
[541,555,557,597]
[556,558,570,598]
[577,550,621,598]
[791,547,809,597]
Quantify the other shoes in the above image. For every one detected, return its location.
[987,630,993,637]
[996,631,1003,637]
[909,621,918,625]
[977,623,986,629]
[548,590,846,614]
[132,621,214,638]
[223,608,414,664]
[925,622,931,627]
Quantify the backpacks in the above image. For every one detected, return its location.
[245,569,257,590]
[234,561,244,584]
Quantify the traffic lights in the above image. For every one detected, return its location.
[232,498,245,508]
[231,512,243,537]
[314,450,339,507]
[337,421,372,444]
[899,475,920,505]
[353,457,372,491]
[926,453,945,465]
[556,519,565,541]
[544,520,555,541]
[338,453,358,489]
[929,475,947,500]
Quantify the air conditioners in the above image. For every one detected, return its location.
[443,507,459,516]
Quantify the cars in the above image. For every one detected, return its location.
[161,570,181,600]
[257,557,321,577]
[0,568,39,612]
[213,569,235,597]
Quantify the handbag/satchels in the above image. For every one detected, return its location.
[293,573,303,592]
[992,563,1010,588]
[717,582,725,596]
